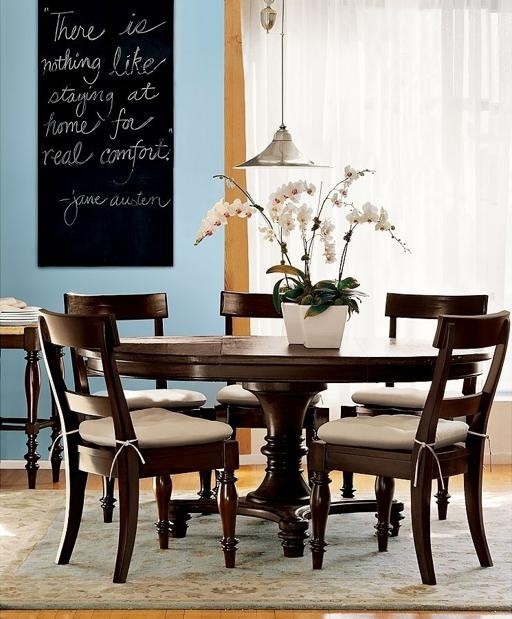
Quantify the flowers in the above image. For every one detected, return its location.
[191,164,412,321]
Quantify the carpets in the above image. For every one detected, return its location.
[0,487,512,611]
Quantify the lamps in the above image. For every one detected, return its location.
[231,0,332,172]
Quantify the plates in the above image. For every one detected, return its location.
[1,306,42,327]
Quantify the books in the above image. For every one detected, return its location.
[0,294,41,327]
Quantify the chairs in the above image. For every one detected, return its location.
[60,291,224,524]
[349,292,491,525]
[210,293,340,513]
[306,310,511,583]
[36,307,239,583]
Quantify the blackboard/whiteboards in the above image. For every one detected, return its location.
[38,0,174,267]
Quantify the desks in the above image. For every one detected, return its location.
[1,323,66,489]
[72,333,490,554]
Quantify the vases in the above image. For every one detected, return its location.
[279,299,350,351]
